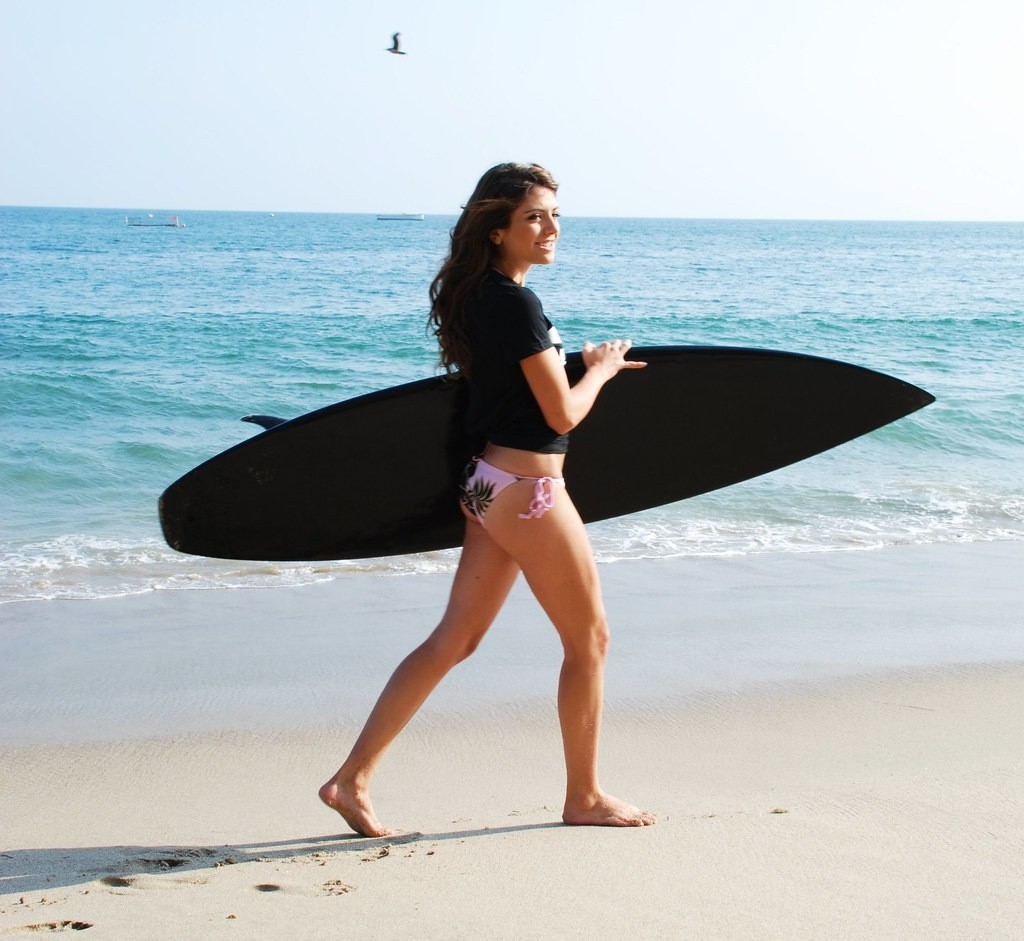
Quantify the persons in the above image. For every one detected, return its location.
[319,162,659,838]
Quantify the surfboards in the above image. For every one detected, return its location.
[156,344,936,562]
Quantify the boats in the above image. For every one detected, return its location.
[124,216,179,226]
[377,213,425,221]
